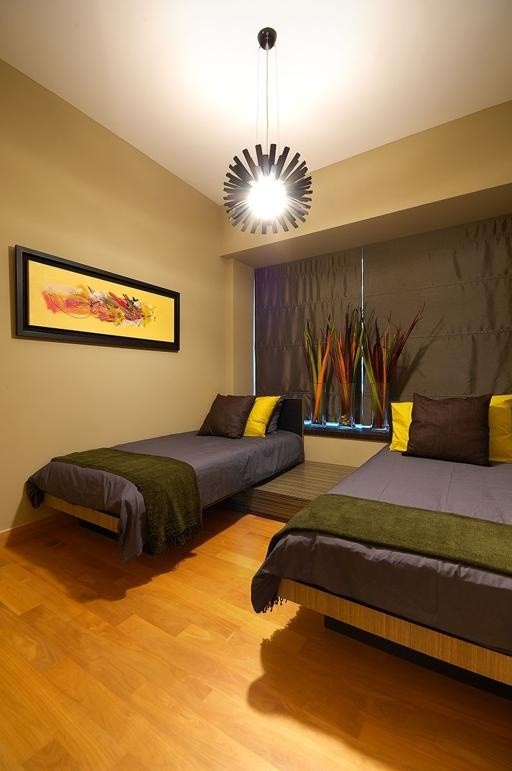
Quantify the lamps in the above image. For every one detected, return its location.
[222,27,313,233]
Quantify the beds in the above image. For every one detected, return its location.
[278,401,512,698]
[43,398,304,543]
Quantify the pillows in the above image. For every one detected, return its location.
[402,393,493,466]
[197,394,255,438]
[244,396,280,438]
[388,394,512,462]
[266,395,285,434]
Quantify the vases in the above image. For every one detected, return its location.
[336,383,355,429]
[368,383,390,433]
[309,382,329,424]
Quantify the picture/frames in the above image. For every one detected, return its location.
[15,244,180,352]
